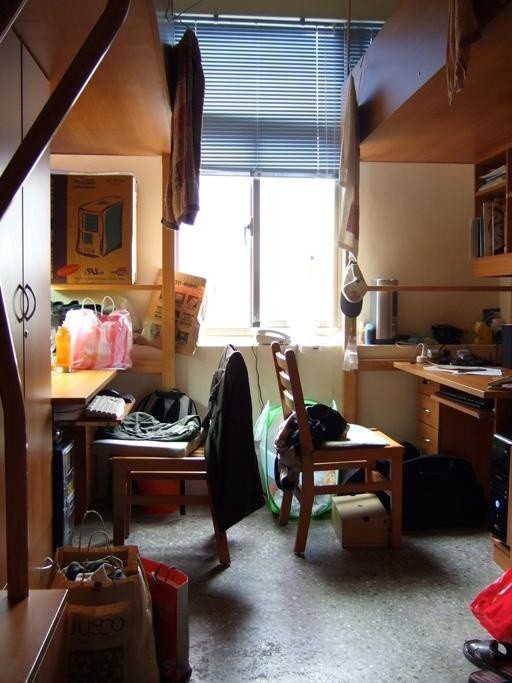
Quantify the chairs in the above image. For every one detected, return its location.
[271,340,406,555]
[106,343,243,567]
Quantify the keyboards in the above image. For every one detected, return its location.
[86,395,125,420]
[439,390,492,409]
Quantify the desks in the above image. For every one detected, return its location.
[50,365,120,550]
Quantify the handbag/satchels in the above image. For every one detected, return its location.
[274,402,348,468]
[375,440,486,539]
[63,297,134,371]
[139,556,192,683]
[48,511,158,682]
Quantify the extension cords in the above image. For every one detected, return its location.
[416,355,451,363]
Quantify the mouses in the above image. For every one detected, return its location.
[98,387,119,397]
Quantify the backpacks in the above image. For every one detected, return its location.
[134,389,199,427]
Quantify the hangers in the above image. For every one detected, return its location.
[164,9,189,46]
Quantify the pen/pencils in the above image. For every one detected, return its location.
[458,369,487,372]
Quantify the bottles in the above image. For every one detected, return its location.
[55,325,72,373]
[470,318,505,345]
[358,320,375,344]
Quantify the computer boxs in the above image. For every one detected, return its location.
[53,436,77,555]
[489,432,512,543]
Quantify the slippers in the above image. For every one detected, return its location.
[463,638,511,683]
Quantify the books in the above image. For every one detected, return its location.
[468,164,506,259]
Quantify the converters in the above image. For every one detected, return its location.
[427,349,440,358]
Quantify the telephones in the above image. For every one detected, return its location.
[256,329,292,345]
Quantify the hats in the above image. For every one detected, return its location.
[340,261,368,319]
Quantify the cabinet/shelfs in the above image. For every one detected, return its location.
[0,19,70,682]
[337,0,512,574]
